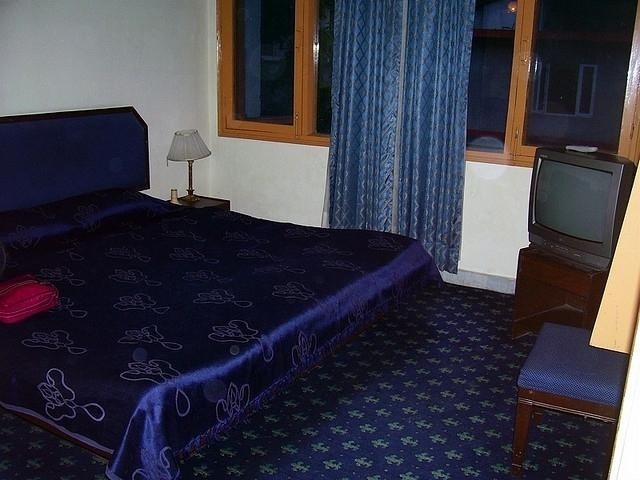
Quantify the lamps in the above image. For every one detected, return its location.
[165,129,212,201]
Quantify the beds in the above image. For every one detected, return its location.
[1,105,431,471]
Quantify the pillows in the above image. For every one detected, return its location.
[0,186,151,258]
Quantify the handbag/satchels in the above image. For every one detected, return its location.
[0,273,61,326]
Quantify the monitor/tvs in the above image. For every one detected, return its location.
[528,146,637,275]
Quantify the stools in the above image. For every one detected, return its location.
[512,321,631,477]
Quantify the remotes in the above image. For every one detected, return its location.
[566,146,598,153]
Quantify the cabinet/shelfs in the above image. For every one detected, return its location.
[511,247,611,349]
